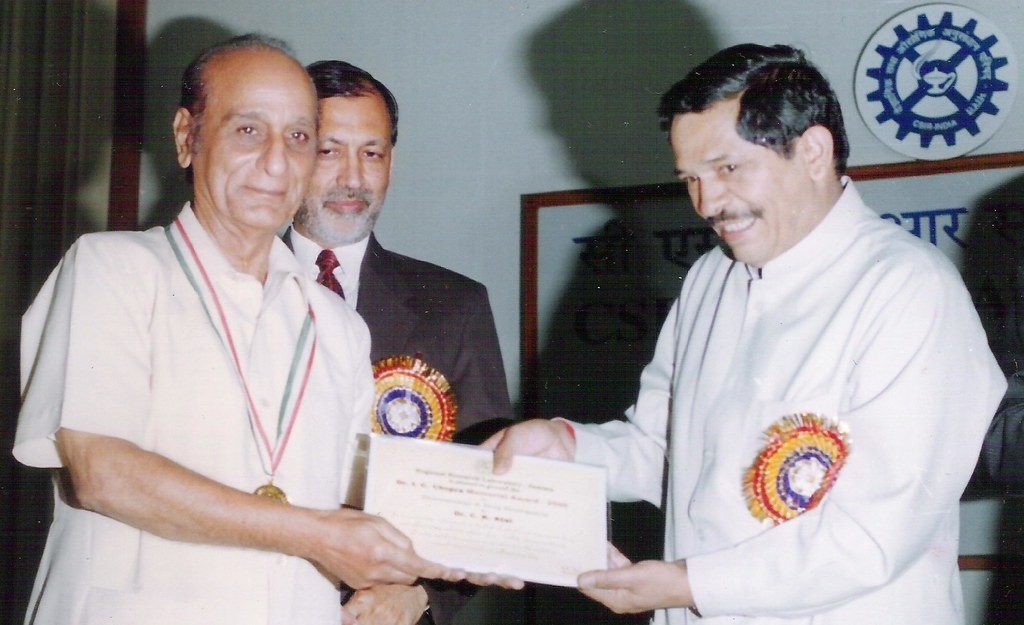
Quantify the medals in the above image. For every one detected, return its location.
[254,484,288,504]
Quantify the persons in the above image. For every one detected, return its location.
[479,40,1009,625]
[12,31,526,625]
[961,232,1024,625]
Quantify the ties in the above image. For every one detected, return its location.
[314,249,346,301]
